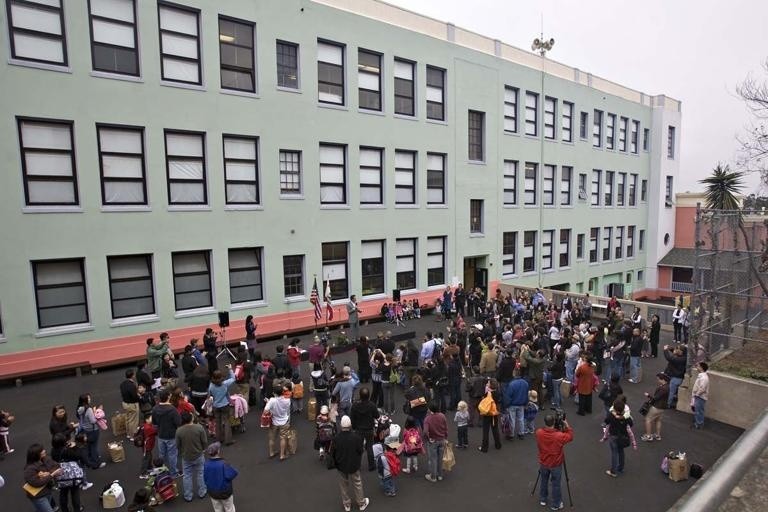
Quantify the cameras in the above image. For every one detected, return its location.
[375,349,379,352]
[225,364,229,367]
[470,326,475,328]
[213,332,217,335]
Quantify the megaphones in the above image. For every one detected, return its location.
[531,38,554,51]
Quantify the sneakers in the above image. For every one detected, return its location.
[345,506,351,512]
[359,497,369,511]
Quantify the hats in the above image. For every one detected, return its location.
[528,390,538,403]
[208,441,221,455]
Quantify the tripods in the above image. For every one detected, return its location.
[216,327,237,360]
[322,346,332,366]
[531,428,573,507]
[390,301,407,327]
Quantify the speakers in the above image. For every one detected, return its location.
[393,290,400,301]
[218,312,229,327]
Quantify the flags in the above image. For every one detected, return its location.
[309,278,323,321]
[324,273,335,322]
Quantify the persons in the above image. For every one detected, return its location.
[244,314,448,508]
[2,327,251,508]
[345,295,363,344]
[379,287,729,510]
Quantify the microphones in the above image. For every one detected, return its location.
[355,303,357,310]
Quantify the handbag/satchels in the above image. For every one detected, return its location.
[55,412,178,509]
[286,384,503,478]
[662,451,703,483]
[640,401,649,417]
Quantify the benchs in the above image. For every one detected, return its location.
[0,358,90,393]
[88,303,437,375]
